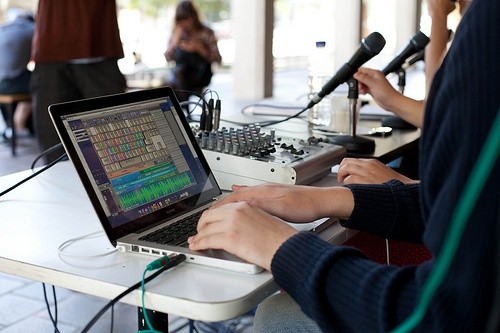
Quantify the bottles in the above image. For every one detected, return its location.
[308,41,334,131]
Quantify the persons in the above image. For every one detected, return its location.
[31,0,126,167]
[186,0,500,333]
[0,14,38,143]
[163,0,222,100]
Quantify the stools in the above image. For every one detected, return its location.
[0,95,28,157]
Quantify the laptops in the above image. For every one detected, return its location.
[49,87,337,275]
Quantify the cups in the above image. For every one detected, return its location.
[331,96,360,132]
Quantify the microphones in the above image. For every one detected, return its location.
[307,32,386,108]
[382,31,429,76]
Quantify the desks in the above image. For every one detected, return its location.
[0,100,425,333]
